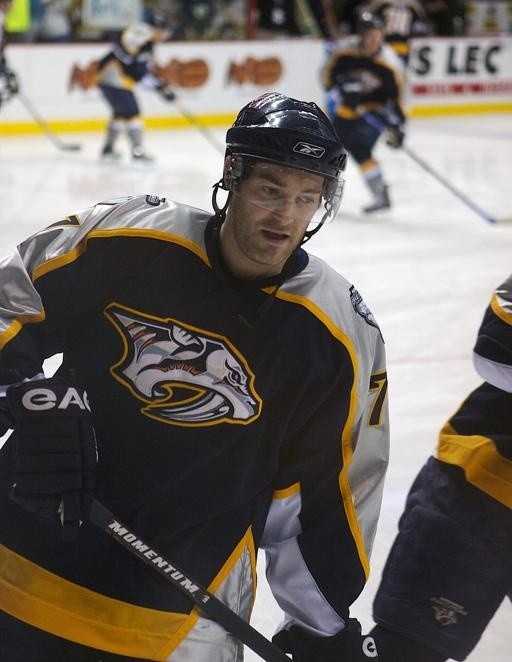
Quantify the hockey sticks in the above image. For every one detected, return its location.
[402,146,511,226]
[15,92,82,152]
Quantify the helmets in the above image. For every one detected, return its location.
[357,12,384,36]
[226,93,347,224]
[146,9,171,28]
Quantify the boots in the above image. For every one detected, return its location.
[362,185,390,213]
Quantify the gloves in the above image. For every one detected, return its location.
[157,80,173,101]
[2,370,98,533]
[386,122,405,146]
[276,618,378,661]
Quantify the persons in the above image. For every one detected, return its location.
[1,1,512,47]
[351,271,512,662]
[316,8,406,218]
[0,18,21,118]
[358,1,433,69]
[92,11,178,167]
[3,88,393,658]
